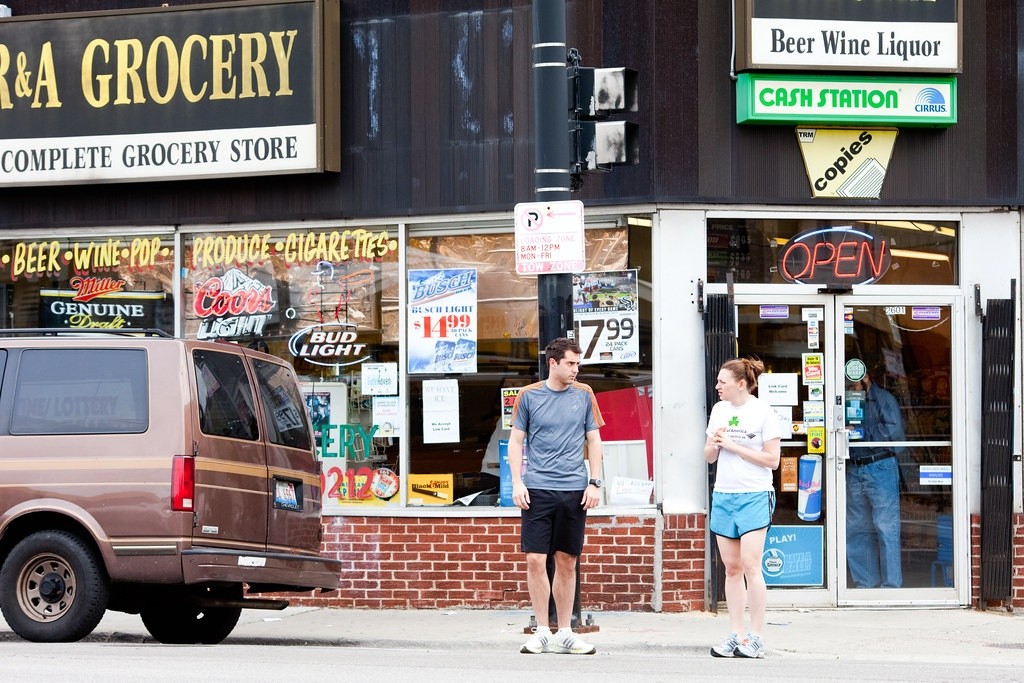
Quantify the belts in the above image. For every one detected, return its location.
[845,453,895,466]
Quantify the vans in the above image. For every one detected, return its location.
[0,326,342,643]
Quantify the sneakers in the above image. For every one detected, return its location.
[710,633,740,658]
[519,627,552,655]
[733,633,765,658]
[552,633,596,654]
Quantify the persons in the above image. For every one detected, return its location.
[508,338,605,655]
[705,356,780,660]
[845,371,906,588]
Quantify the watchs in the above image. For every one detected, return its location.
[588,477,600,488]
